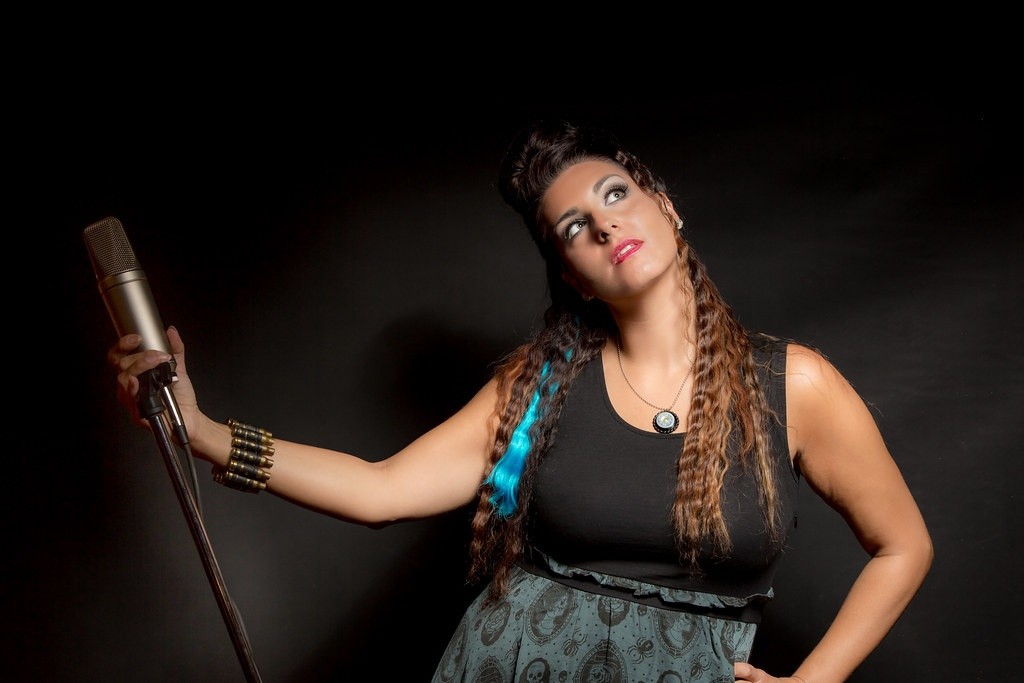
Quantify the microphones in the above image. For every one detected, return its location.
[85,216,191,444]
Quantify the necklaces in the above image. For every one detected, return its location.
[616,334,697,434]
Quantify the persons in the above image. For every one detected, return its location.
[109,125,934,683]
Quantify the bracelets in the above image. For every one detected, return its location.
[211,416,275,494]
